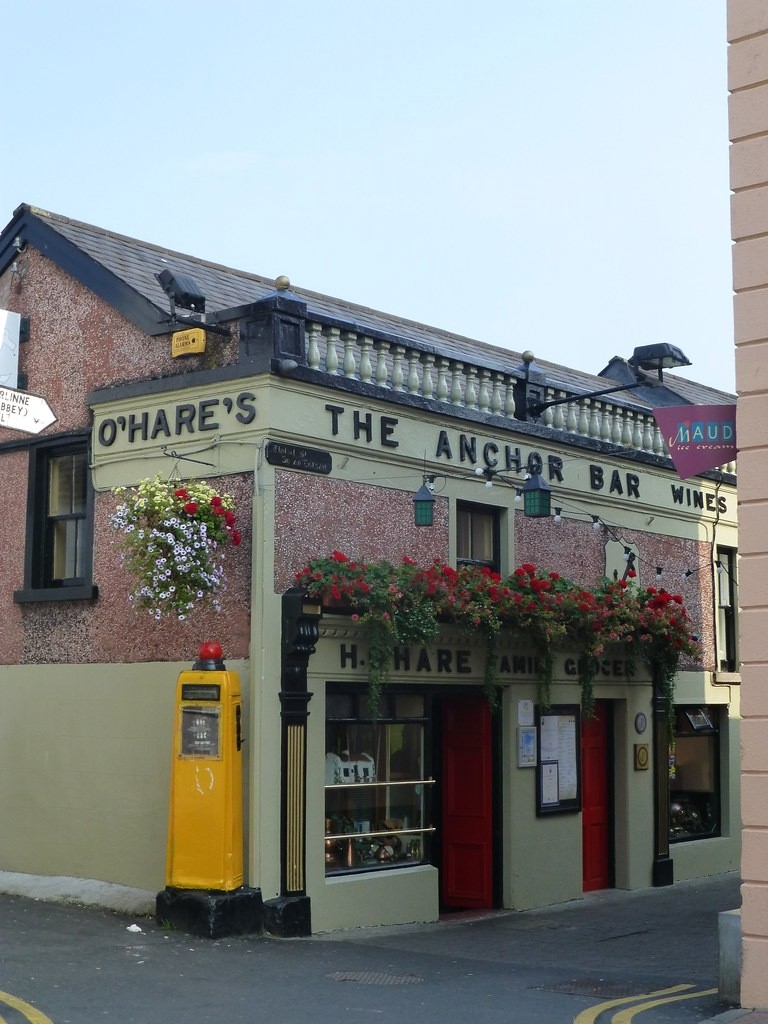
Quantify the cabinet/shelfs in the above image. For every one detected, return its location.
[321,717,427,877]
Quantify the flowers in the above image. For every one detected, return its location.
[496,562,573,717]
[635,585,696,751]
[293,549,434,731]
[108,472,242,622]
[434,557,502,715]
[573,569,637,722]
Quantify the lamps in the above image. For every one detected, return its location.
[521,470,553,518]
[532,341,693,418]
[12,237,27,254]
[154,268,230,338]
[412,481,435,527]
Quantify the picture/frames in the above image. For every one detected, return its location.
[516,727,537,768]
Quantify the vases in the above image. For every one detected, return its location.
[322,587,351,608]
